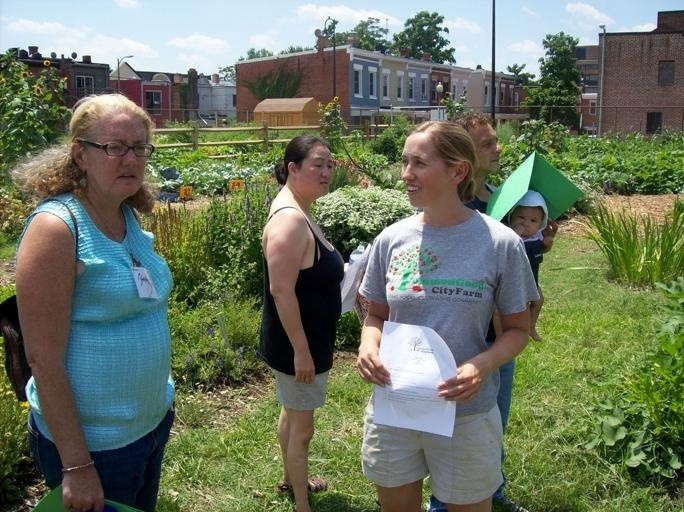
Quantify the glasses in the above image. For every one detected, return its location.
[72,137,155,158]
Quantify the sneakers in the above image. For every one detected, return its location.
[279,477,327,493]
[492,491,529,512]
[428,499,448,512]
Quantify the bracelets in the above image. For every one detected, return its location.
[59,460,99,472]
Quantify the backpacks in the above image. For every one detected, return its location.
[0,297,38,403]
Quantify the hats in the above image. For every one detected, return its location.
[508,189,549,236]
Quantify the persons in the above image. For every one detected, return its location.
[254,135,346,512]
[427,112,558,511]
[491,187,553,342]
[354,119,540,511]
[14,91,203,510]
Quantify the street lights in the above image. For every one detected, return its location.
[596,23,606,137]
[322,18,338,100]
[434,81,443,121]
[116,55,132,93]
